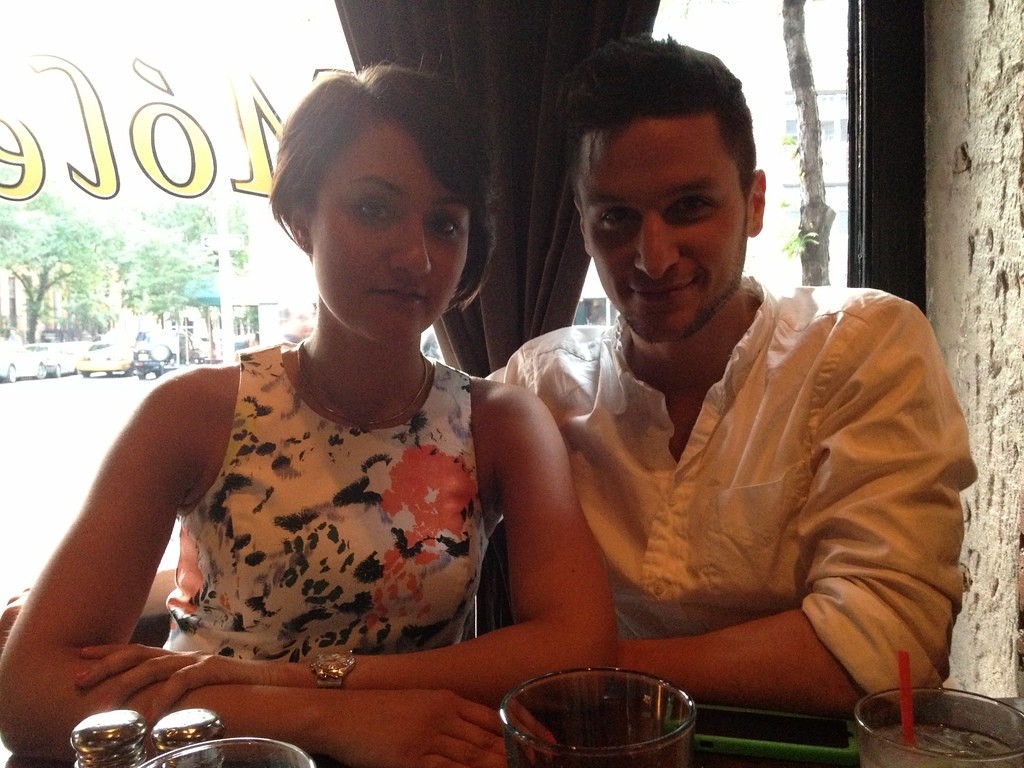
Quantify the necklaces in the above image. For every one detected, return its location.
[296,338,429,426]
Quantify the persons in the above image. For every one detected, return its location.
[1,66,618,768]
[0,36,979,720]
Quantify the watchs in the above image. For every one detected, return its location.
[309,653,355,688]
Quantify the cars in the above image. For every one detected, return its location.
[0,334,255,380]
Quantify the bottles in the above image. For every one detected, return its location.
[69,709,147,768]
[151,707,227,768]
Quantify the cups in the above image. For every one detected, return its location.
[854,687,1024,768]
[139,737,316,768]
[500,667,696,768]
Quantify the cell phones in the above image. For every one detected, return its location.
[694,704,861,766]
